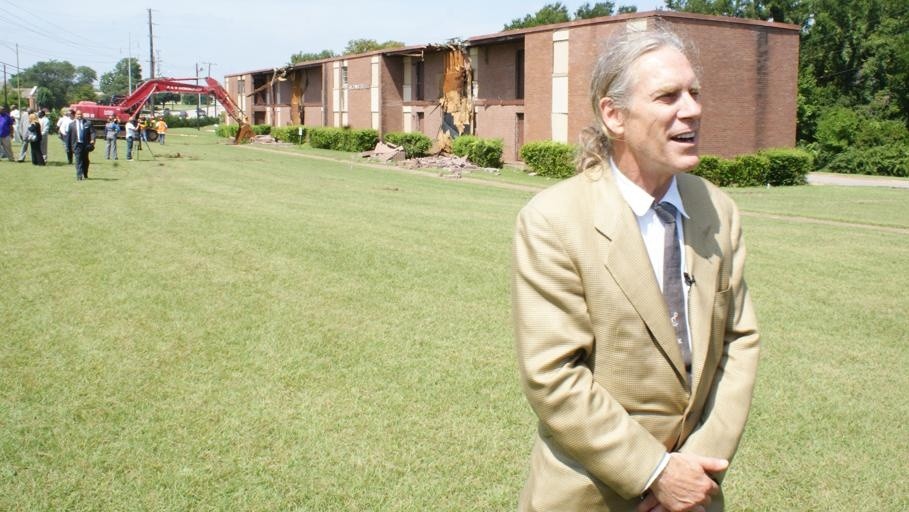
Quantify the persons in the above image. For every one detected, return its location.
[513,23,760,512]
[0,101,167,180]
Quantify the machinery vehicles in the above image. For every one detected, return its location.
[63,73,254,145]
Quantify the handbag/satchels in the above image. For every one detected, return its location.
[23,130,38,143]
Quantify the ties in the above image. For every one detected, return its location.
[78,120,84,144]
[650,202,693,396]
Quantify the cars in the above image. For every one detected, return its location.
[138,106,206,121]
